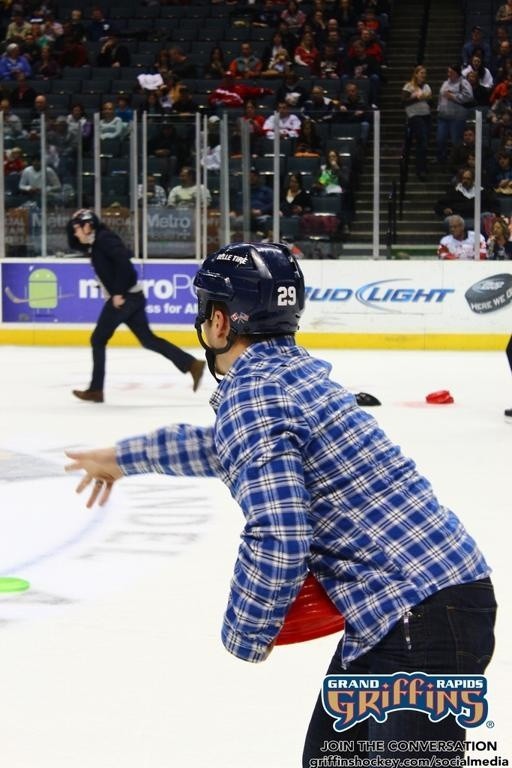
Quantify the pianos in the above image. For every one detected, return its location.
[3,286,74,304]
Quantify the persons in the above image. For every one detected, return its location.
[504,336,512,416]
[63,243,499,767]
[72,207,205,402]
[0,0,512,258]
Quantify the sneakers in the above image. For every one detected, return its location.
[188,360,206,392]
[73,387,104,402]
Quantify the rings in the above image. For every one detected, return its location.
[96,480,104,485]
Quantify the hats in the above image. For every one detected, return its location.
[449,60,463,76]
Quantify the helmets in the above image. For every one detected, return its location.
[70,209,99,229]
[193,242,304,334]
